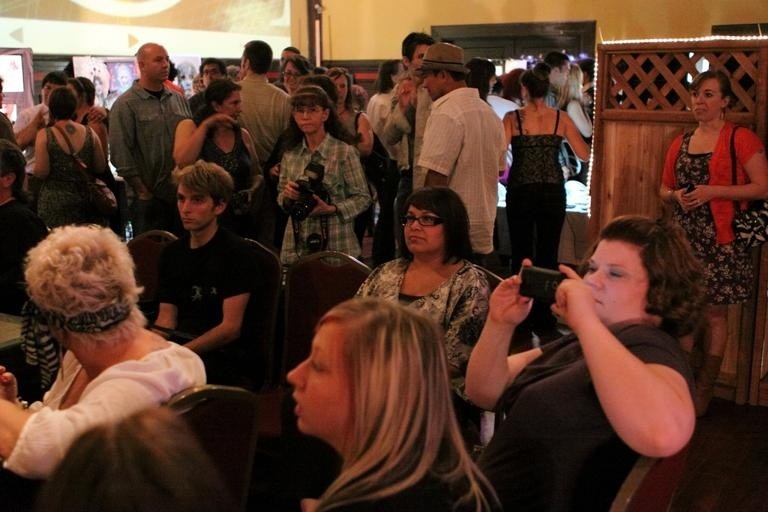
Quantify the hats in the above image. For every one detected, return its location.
[413,42,471,75]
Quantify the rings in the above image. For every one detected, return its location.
[697,198,700,204]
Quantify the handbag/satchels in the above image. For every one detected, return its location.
[731,199,768,249]
[88,175,120,210]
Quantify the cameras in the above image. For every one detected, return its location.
[291,162,328,221]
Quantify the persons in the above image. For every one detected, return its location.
[2,29,591,318]
[286,297,503,512]
[149,157,263,391]
[0,220,206,482]
[460,213,707,511]
[352,183,503,450]
[658,68,767,416]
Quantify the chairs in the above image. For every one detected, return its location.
[237,238,284,394]
[127,231,182,309]
[278,249,374,388]
[162,385,261,511]
[472,262,503,297]
[607,454,682,511]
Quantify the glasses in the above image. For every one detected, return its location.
[293,106,325,113]
[400,214,448,226]
[281,70,305,77]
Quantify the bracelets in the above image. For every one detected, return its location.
[667,188,673,202]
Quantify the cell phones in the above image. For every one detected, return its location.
[519,265,567,301]
[687,183,702,211]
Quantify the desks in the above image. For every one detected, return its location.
[494,190,590,270]
